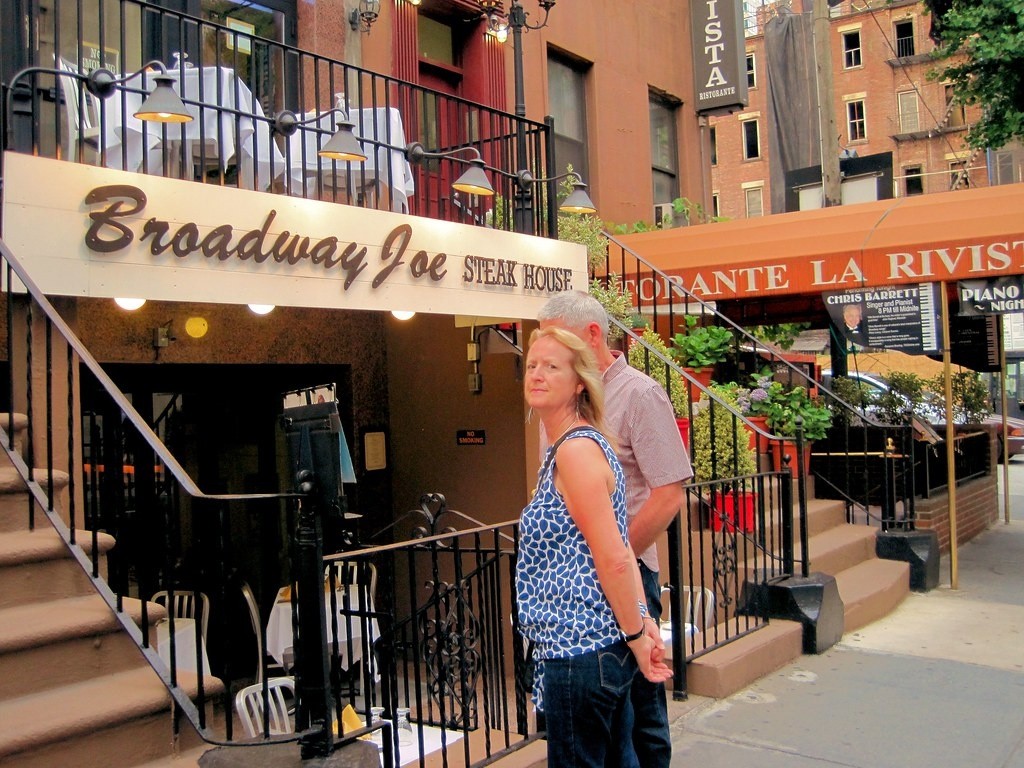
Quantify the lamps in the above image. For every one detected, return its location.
[837,134,858,160]
[403,142,496,197]
[496,19,508,43]
[515,169,597,214]
[84,60,193,123]
[276,107,369,161]
[348,0,380,36]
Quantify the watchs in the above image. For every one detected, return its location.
[620,627,644,641]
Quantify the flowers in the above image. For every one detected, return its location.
[735,372,784,416]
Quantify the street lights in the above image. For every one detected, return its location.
[477,0,557,235]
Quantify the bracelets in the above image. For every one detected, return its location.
[642,616,656,623]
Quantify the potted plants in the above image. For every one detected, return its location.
[667,313,733,402]
[631,334,689,458]
[627,314,649,358]
[771,387,831,476]
[694,390,757,535]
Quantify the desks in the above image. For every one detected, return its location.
[659,619,700,649]
[355,718,465,768]
[89,66,286,192]
[265,584,382,696]
[154,617,213,680]
[285,102,416,213]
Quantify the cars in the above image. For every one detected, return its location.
[818,371,1024,463]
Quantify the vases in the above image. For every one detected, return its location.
[739,416,772,454]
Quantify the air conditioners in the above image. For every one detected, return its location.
[654,204,673,231]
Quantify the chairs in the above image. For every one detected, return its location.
[323,558,376,605]
[659,583,715,632]
[234,582,296,740]
[151,590,210,647]
[51,50,104,169]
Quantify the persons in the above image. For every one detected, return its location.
[515,327,673,768]
[539,291,693,768]
[843,305,865,347]
